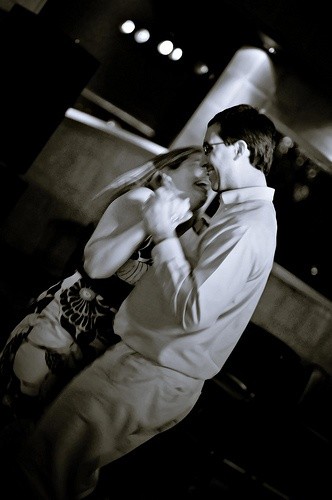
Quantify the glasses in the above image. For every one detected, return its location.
[202,140,236,156]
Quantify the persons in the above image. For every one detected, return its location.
[0,104,277,500]
